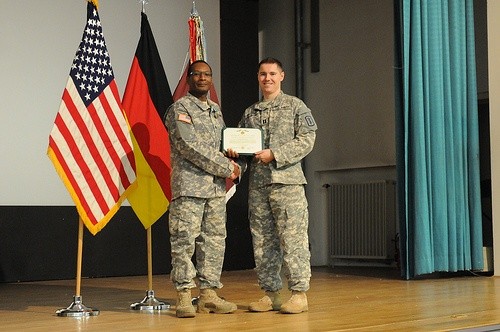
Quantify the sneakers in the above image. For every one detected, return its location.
[197,288,237,314]
[175,290,196,317]
[280,291,309,313]
[249,291,281,312]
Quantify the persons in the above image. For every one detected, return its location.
[222,57,318,314]
[164,60,248,317]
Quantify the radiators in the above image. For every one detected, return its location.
[323,179,400,272]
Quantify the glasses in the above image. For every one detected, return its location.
[189,71,211,77]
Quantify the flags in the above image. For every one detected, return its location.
[47,1,237,236]
